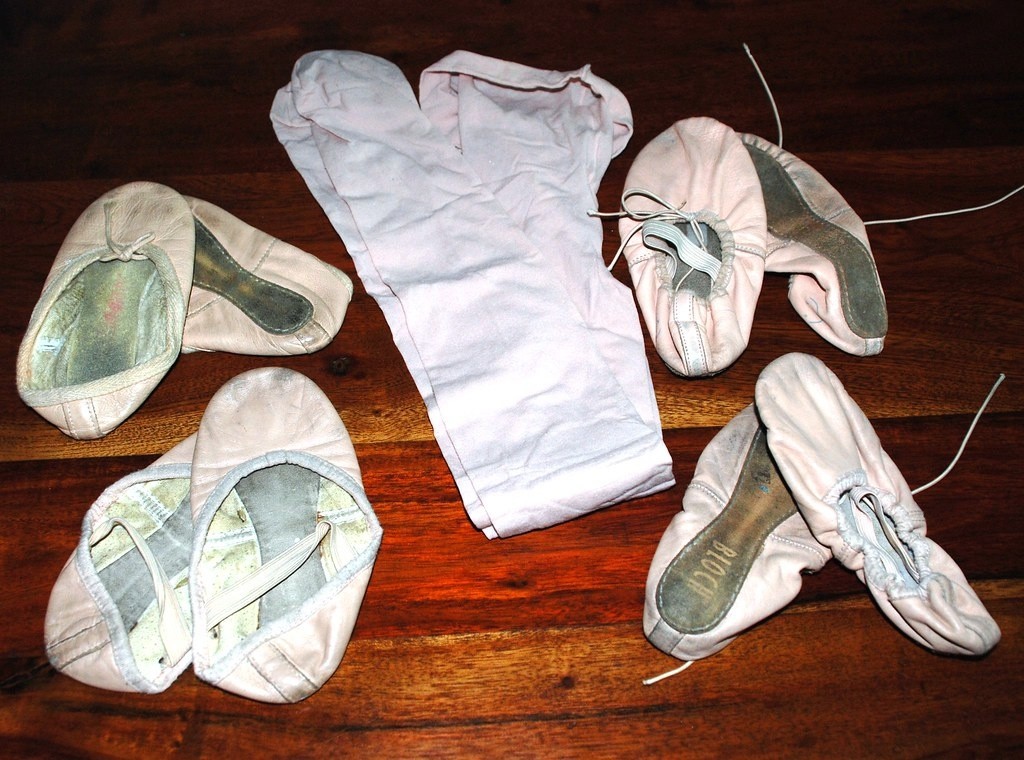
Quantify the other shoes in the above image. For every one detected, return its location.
[16,182,196,441]
[583,116,765,377]
[177,195,354,355]
[754,351,1005,654]
[190,368,383,706]
[640,405,835,685]
[724,42,1024,357]
[45,431,202,695]
